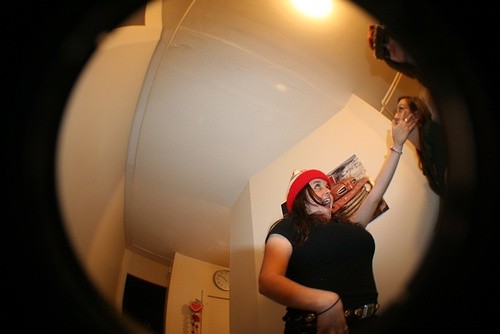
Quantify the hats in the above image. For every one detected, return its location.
[286,169,333,212]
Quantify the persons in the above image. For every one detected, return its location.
[258,112,418,334]
[394,96,457,195]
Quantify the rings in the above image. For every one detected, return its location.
[403,119,408,122]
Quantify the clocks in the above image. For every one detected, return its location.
[213,270,230,291]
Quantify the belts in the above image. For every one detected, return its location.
[288,304,380,323]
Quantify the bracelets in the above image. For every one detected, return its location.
[318,297,341,316]
[389,146,402,155]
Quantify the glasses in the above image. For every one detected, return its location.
[392,105,409,115]
